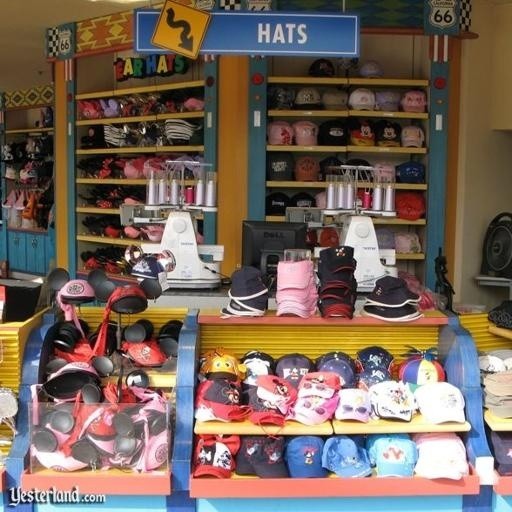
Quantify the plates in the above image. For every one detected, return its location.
[264,77,429,260]
[75,78,205,285]
[5,103,55,277]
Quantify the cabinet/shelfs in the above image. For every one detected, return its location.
[264,77,429,260]
[3,303,512,512]
[75,78,205,285]
[5,103,55,277]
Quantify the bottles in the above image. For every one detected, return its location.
[220,227,438,322]
[266,57,427,222]
[30,268,183,473]
[192,346,470,481]
[75,92,205,279]
[478,349,512,477]
